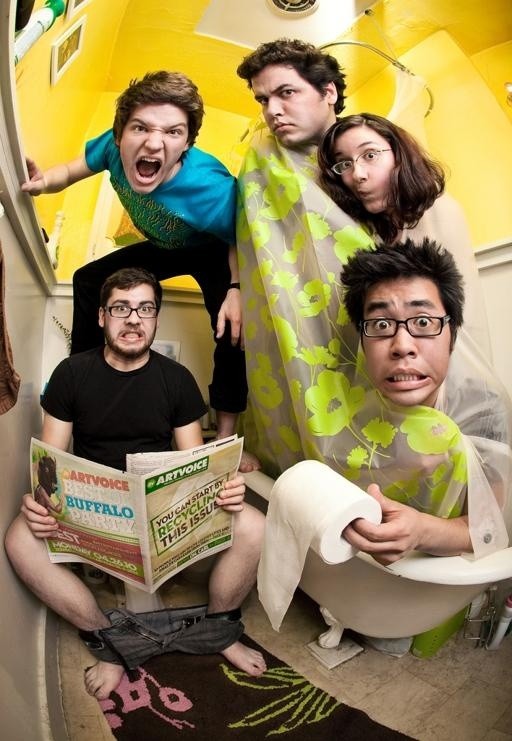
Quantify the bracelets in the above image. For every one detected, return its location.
[226,281,241,290]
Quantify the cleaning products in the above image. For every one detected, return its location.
[488,594,512,650]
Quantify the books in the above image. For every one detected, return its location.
[23,431,246,593]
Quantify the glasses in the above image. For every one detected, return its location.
[104,303,161,321]
[360,313,452,339]
[329,147,397,177]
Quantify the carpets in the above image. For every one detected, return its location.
[85,630,421,741]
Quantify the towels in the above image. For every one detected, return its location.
[0,238,23,414]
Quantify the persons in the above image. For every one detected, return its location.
[234,37,348,150]
[25,70,261,476]
[338,239,512,569]
[3,268,267,701]
[317,110,447,244]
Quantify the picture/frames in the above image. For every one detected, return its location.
[63,0,92,24]
[50,14,87,86]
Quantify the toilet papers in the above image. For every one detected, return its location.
[257,458,382,634]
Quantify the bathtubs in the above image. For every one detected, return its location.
[237,432,512,648]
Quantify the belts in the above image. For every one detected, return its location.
[79,609,245,643]
